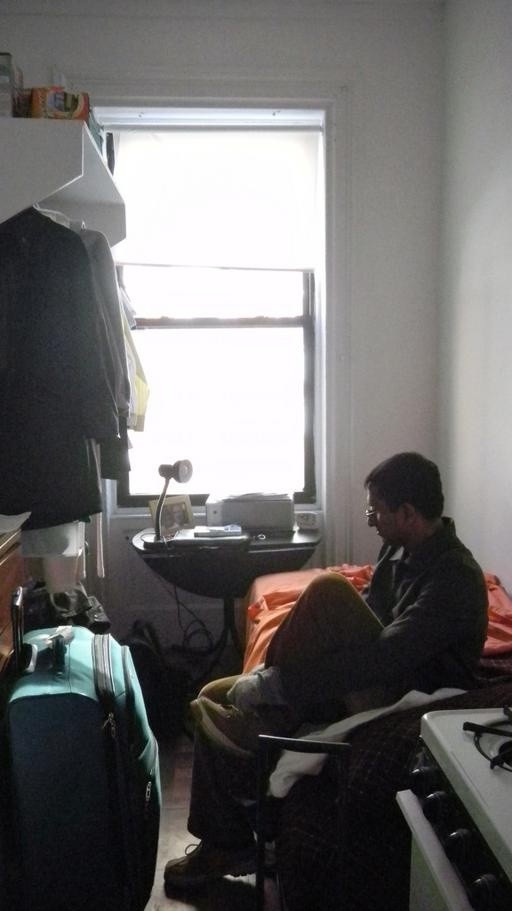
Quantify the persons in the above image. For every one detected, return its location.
[164,450,490,888]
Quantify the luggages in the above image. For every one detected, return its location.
[4,585,162,910]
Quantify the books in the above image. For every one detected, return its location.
[194,523,242,537]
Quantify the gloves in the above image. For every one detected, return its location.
[226,674,268,714]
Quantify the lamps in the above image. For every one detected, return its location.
[142,458,194,550]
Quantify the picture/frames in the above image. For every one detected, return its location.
[148,494,194,537]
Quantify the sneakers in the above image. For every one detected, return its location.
[189,696,262,762]
[164,839,265,888]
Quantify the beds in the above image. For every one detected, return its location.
[241,564,511,720]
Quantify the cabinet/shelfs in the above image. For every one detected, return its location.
[0,540,27,679]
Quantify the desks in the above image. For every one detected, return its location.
[135,529,322,664]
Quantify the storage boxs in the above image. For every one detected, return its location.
[204,490,296,534]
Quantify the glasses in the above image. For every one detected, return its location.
[365,509,377,521]
